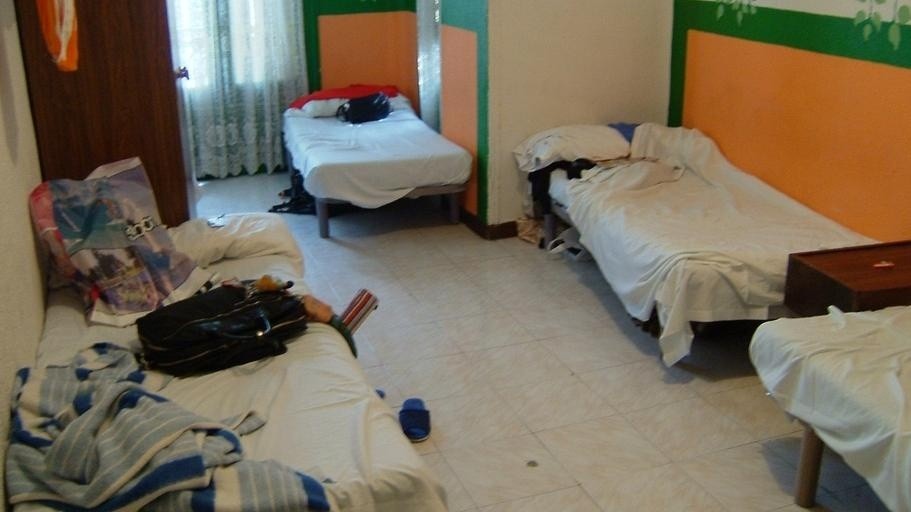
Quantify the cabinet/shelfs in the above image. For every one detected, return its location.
[14,0,198,226]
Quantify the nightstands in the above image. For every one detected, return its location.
[783,240,911,313]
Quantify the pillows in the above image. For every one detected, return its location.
[301,94,413,117]
[167,214,304,278]
[511,122,631,171]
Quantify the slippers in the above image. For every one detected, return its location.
[399,400,430,441]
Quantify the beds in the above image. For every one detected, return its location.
[525,121,886,366]
[282,93,474,237]
[747,306,911,512]
[0,213,448,512]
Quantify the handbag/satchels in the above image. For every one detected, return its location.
[136,280,305,375]
[337,93,389,124]
